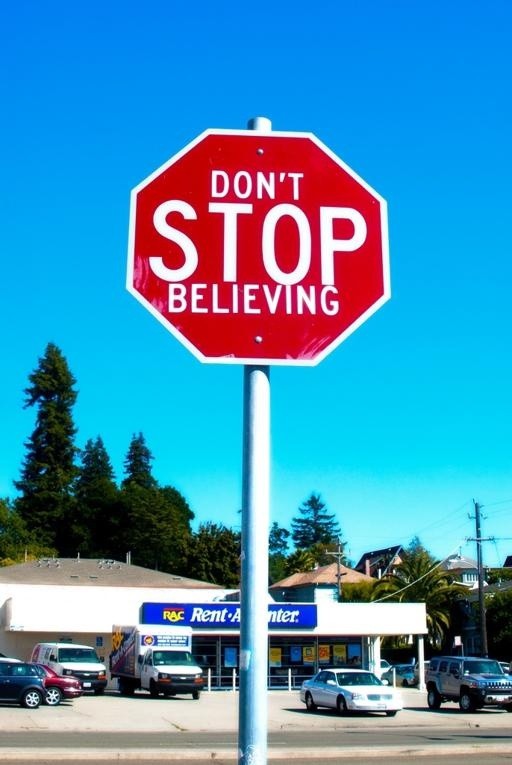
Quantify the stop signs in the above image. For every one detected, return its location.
[125,128,391,368]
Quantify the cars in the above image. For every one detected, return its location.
[0,658,83,709]
[379,656,425,714]
[300,668,403,718]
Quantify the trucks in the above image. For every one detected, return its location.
[110,625,206,700]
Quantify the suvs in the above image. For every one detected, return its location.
[425,656,512,713]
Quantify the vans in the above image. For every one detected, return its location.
[31,643,107,695]
